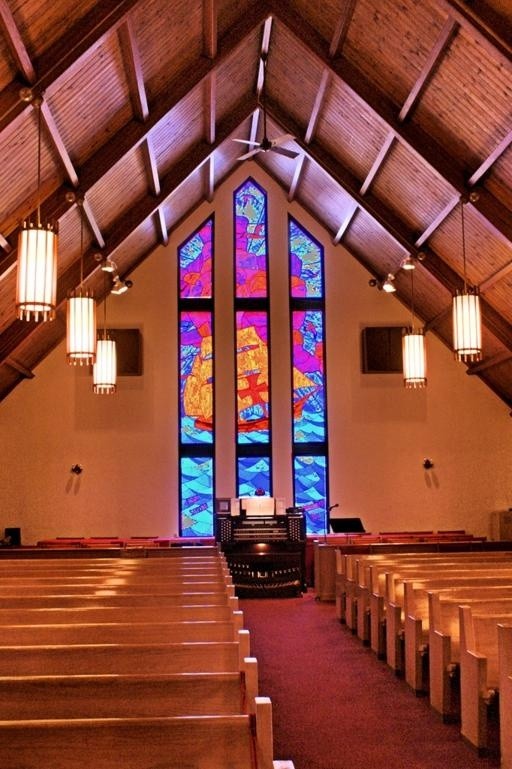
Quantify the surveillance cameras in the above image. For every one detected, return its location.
[369,279,377,287]
[125,280,133,288]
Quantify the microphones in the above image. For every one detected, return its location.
[329,503,339,511]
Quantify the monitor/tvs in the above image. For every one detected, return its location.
[329,518,366,533]
[216,498,231,514]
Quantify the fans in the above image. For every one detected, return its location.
[231,51,306,163]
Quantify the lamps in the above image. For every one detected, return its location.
[16,94,130,396]
[383,192,482,387]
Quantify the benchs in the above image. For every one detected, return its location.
[0,536,275,769]
[302,528,511,768]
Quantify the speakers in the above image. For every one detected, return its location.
[361,325,413,373]
[89,328,143,377]
